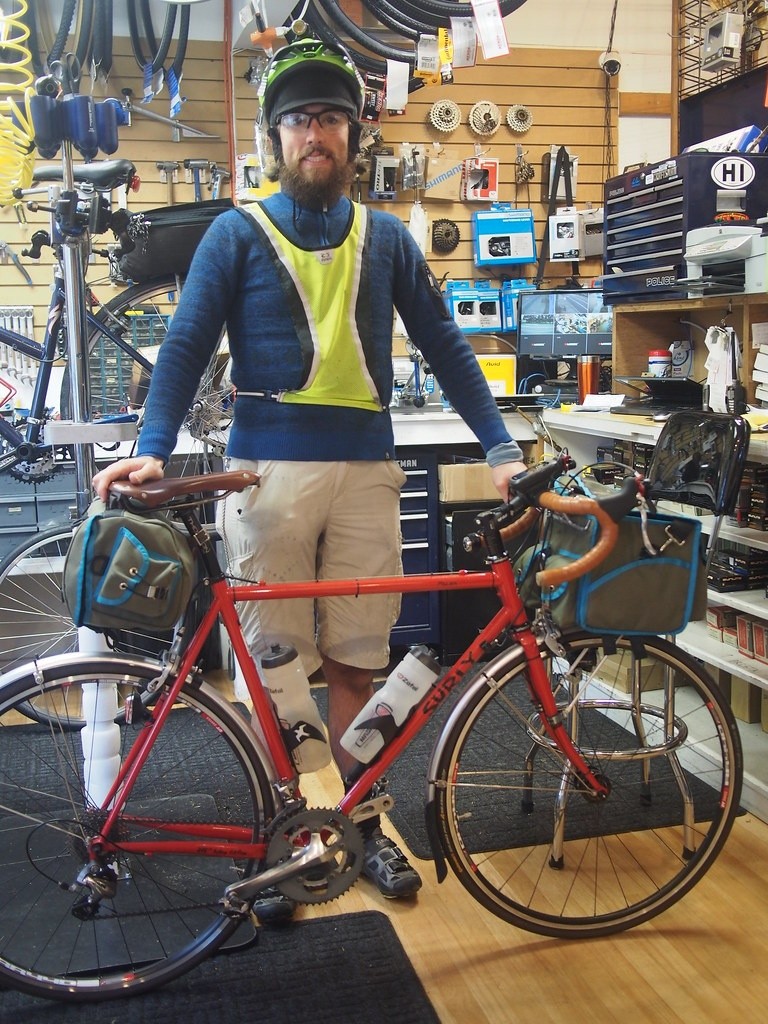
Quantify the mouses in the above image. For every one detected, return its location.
[653,411,673,421]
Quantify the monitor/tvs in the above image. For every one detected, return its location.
[516,289,613,386]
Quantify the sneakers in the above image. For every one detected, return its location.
[349,827,422,898]
[253,897,296,923]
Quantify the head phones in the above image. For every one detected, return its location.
[255,40,365,182]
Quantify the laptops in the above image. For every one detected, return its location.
[610,375,705,416]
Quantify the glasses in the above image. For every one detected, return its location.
[279,109,353,132]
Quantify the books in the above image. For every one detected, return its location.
[752,344,768,404]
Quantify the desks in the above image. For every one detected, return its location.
[92,409,541,463]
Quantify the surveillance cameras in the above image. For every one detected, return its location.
[602,51,621,76]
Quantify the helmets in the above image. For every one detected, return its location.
[257,39,366,129]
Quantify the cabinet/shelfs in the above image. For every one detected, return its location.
[537,295,768,821]
[383,451,443,668]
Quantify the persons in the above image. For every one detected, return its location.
[83,34,534,898]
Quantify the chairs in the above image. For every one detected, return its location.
[513,407,755,871]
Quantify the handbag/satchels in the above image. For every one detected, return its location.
[117,197,233,284]
[61,498,195,632]
[514,506,707,636]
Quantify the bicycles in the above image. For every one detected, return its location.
[1,451,746,1003]
[0,153,237,505]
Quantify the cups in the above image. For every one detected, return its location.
[576,355,600,405]
[648,349,672,378]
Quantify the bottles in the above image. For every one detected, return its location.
[261,642,332,773]
[339,644,441,765]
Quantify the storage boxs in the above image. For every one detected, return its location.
[0,14,768,731]
